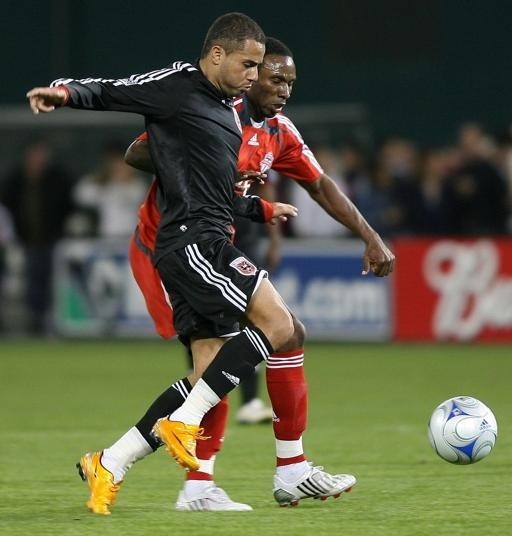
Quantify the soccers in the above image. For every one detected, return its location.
[427,396,498,465]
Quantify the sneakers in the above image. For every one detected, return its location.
[76,450,125,516]
[273,465,357,508]
[149,416,211,473]
[175,484,253,512]
[236,397,272,425]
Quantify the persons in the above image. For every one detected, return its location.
[287,123,512,239]
[25,14,299,515]
[1,138,76,344]
[182,168,283,425]
[73,152,151,236]
[122,35,396,512]
